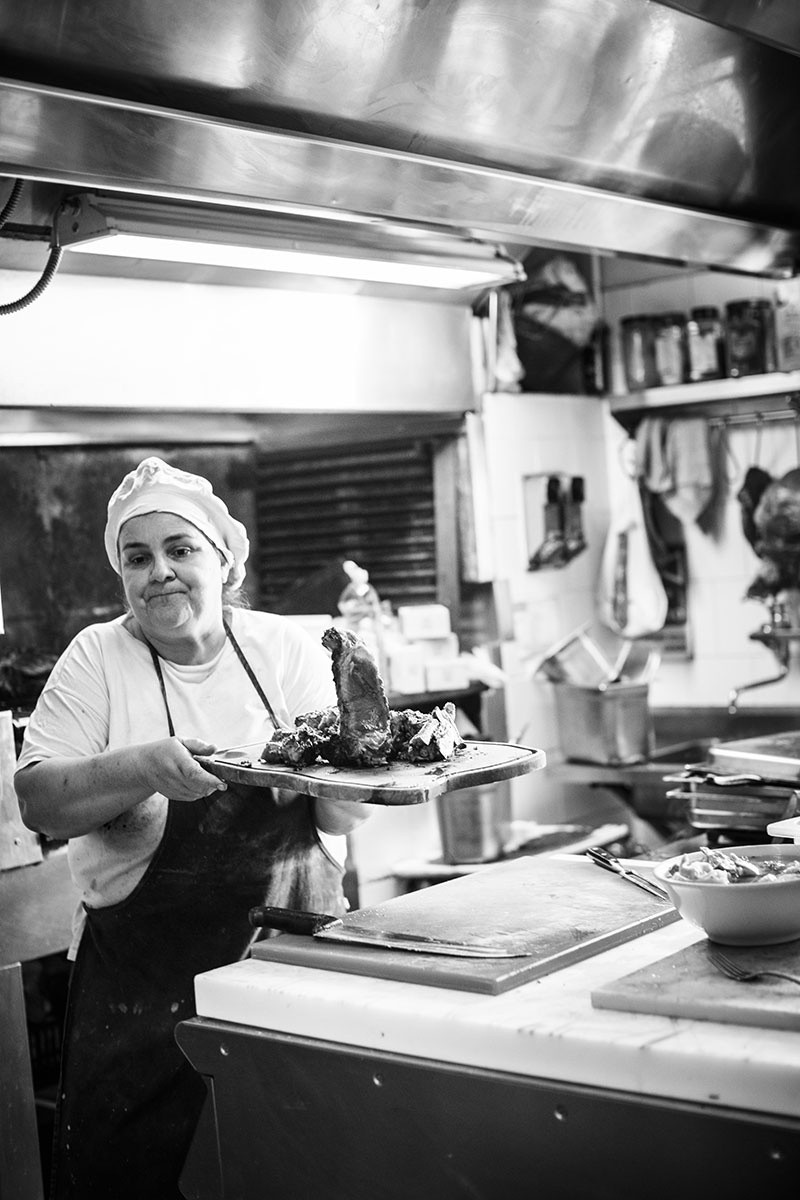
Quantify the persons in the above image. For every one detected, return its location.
[13,454,374,1200]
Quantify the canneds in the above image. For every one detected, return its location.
[620,297,783,392]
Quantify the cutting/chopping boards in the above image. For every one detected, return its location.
[251,855,683,997]
[590,937,800,1030]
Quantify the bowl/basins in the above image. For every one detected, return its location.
[653,844,800,945]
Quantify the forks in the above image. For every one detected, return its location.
[707,951,800,985]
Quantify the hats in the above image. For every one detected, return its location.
[105,457,250,596]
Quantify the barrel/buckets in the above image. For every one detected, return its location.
[436,779,513,865]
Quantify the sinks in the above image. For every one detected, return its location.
[623,736,724,817]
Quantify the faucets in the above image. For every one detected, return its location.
[727,644,790,713]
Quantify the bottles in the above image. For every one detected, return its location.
[653,312,686,386]
[620,310,657,391]
[687,305,725,383]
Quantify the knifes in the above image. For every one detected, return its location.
[248,906,532,958]
